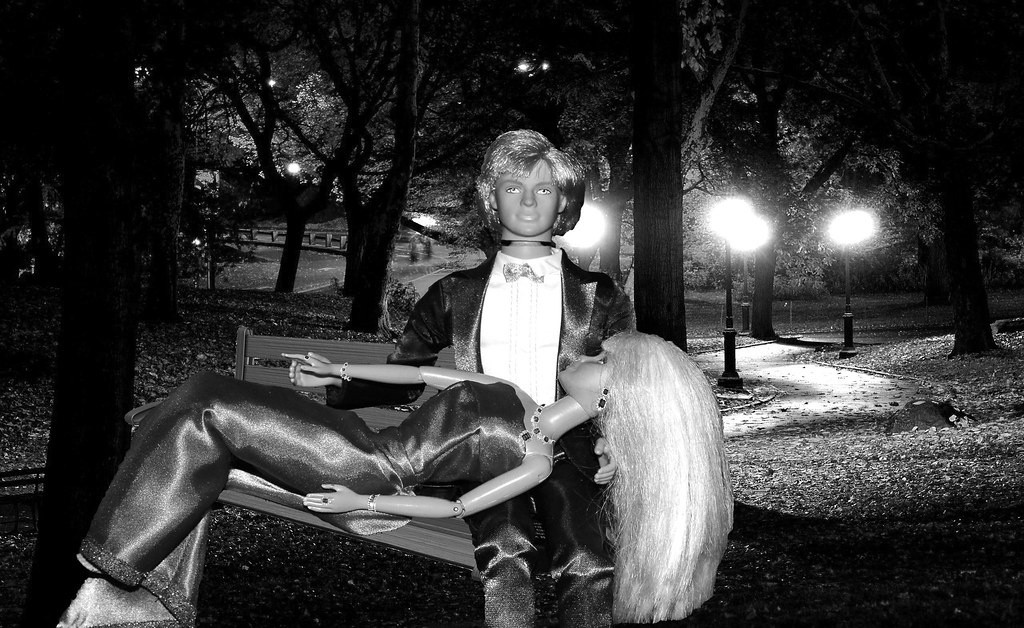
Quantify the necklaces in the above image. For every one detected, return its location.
[501,238,555,247]
[519,405,556,455]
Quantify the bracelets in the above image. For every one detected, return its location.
[340,362,351,381]
[368,494,379,511]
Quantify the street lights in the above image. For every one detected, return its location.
[739,219,759,336]
[715,201,743,388]
[834,213,861,359]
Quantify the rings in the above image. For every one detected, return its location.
[304,355,310,360]
[322,498,328,503]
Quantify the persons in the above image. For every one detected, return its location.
[282,129,637,628]
[56,332,734,628]
